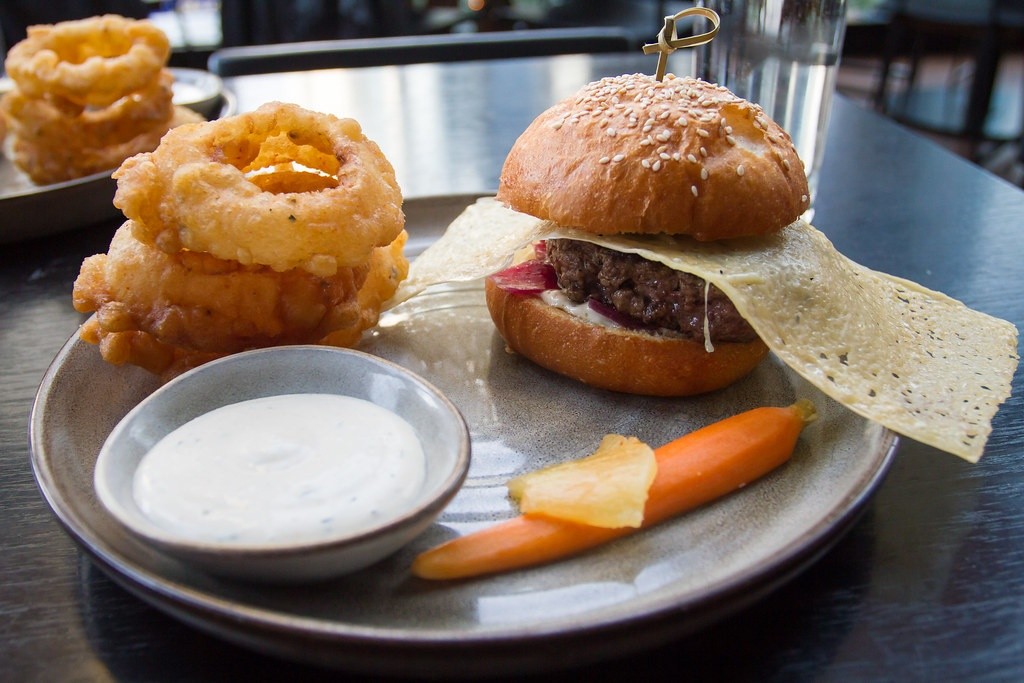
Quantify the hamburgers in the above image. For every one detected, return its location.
[384,74,1022,463]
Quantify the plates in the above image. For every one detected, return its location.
[0,69,237,245]
[92,344,472,583]
[29,190,899,679]
[165,67,223,116]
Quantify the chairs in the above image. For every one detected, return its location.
[207,17,696,81]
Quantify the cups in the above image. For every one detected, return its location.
[690,1,846,226]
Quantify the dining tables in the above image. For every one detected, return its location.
[867,1,1024,141]
[0,49,1023,682]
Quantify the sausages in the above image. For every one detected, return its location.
[413,397,815,576]
